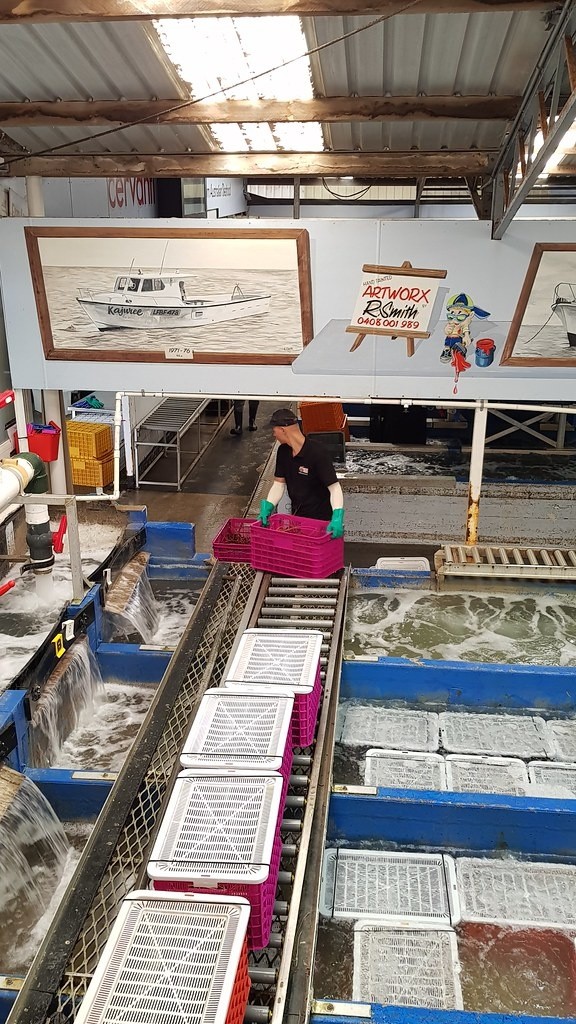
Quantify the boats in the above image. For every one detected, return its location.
[76,241,271,329]
[550,281,576,348]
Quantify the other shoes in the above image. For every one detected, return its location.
[249,426,258,431]
[229,428,243,436]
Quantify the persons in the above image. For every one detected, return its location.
[229,400,260,434]
[256,409,344,540]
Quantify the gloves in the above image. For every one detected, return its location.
[84,395,104,408]
[327,508,345,539]
[256,500,275,527]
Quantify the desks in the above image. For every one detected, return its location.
[134,397,234,492]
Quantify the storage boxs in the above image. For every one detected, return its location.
[212,517,258,562]
[66,412,126,486]
[251,515,343,579]
[68,628,576,1023]
[299,401,350,440]
